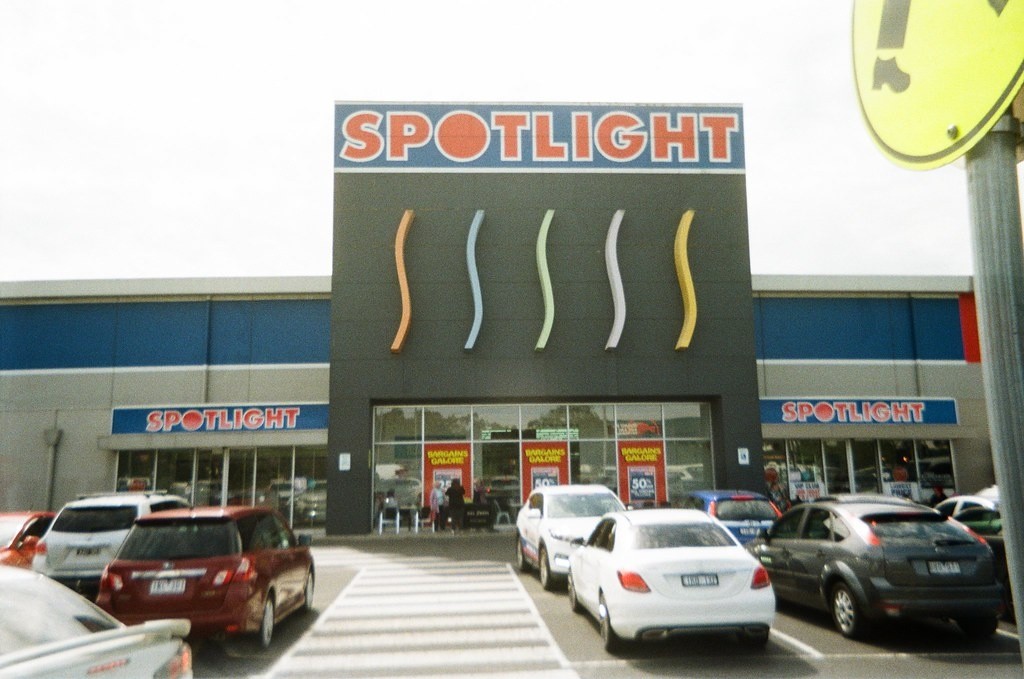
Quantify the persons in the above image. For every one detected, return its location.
[769,482,784,511]
[429,481,446,532]
[470,477,497,532]
[385,489,402,519]
[445,478,466,534]
[927,486,948,508]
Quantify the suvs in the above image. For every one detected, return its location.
[32,491,192,597]
[516,485,634,589]
[97,506,316,648]
[672,492,780,544]
[483,476,522,504]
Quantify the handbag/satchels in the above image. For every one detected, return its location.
[430,510,437,522]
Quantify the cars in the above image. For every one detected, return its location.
[0,512,56,569]
[376,477,423,506]
[568,508,775,654]
[783,458,954,486]
[1,565,194,679]
[121,477,327,522]
[743,491,1004,637]
[577,464,704,494]
[933,494,1004,537]
